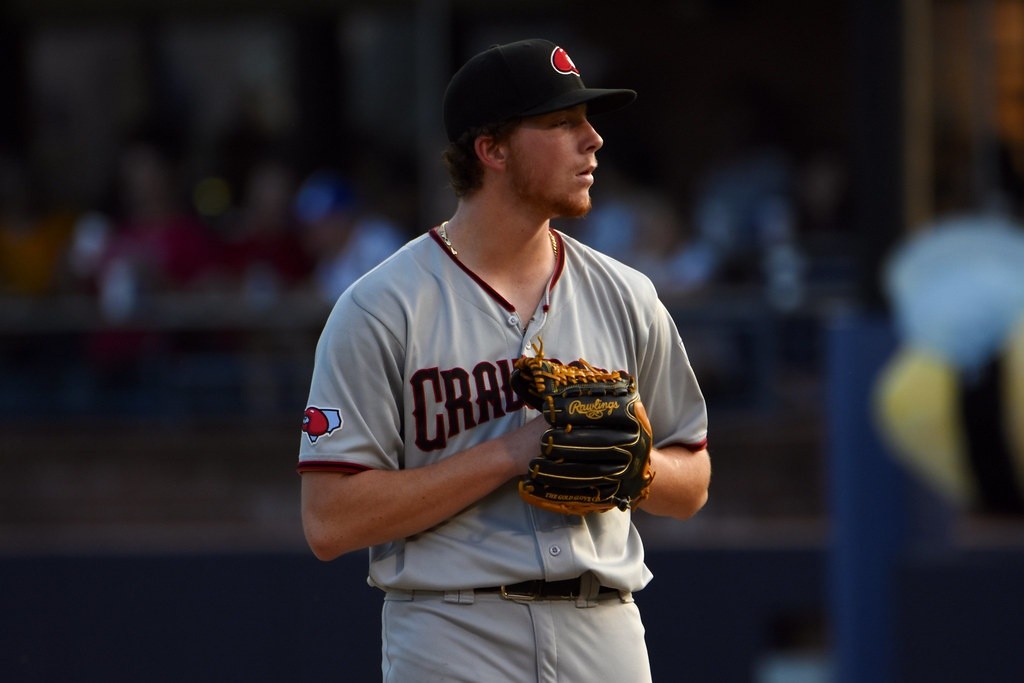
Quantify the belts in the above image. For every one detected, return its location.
[473,577,618,600]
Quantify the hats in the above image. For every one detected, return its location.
[442,38,635,142]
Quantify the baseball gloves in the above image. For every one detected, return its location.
[510,336,656,516]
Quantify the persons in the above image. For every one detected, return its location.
[296,38,712,683]
